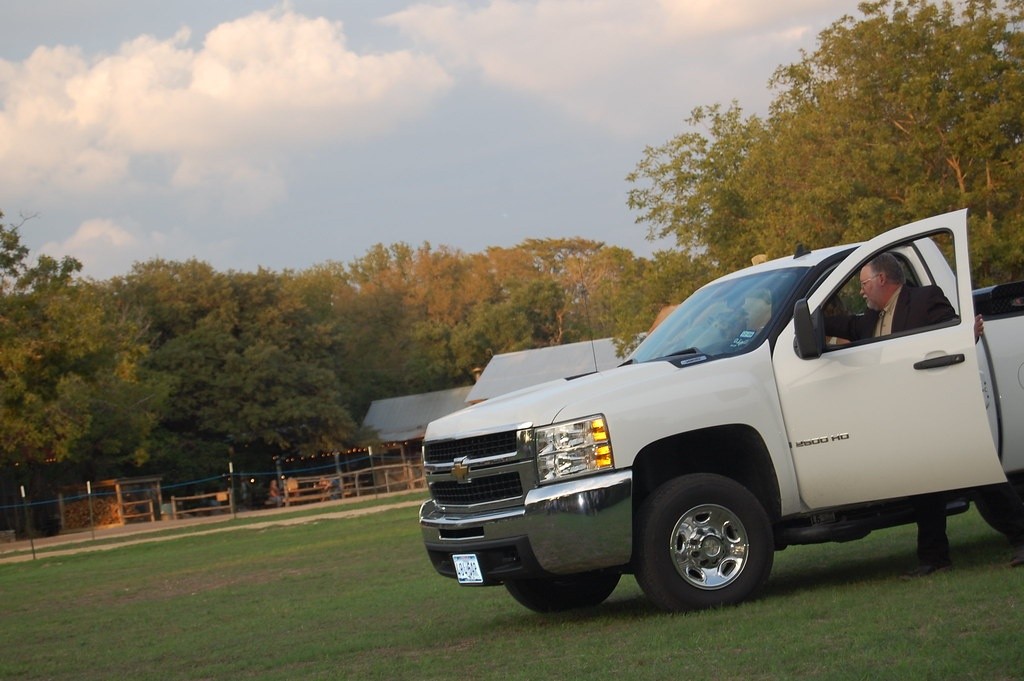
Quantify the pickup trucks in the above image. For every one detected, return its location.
[417,208,1024,612]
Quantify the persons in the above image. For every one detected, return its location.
[315,477,340,499]
[820,252,1024,575]
[270,480,281,507]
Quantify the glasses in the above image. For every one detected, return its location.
[859,272,880,289]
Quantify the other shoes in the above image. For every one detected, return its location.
[1010,548,1024,567]
[901,564,950,584]
